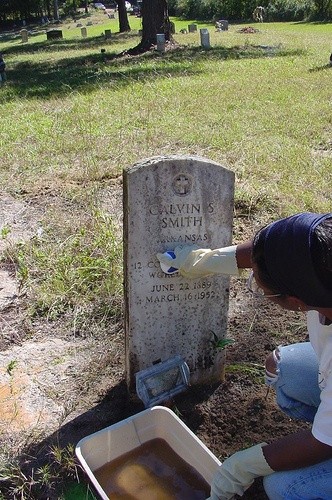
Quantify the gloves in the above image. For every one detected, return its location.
[157,244,243,279]
[210,443,275,500]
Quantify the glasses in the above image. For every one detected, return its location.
[248,273,281,299]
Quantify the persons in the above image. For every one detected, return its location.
[156,212,332,500]
